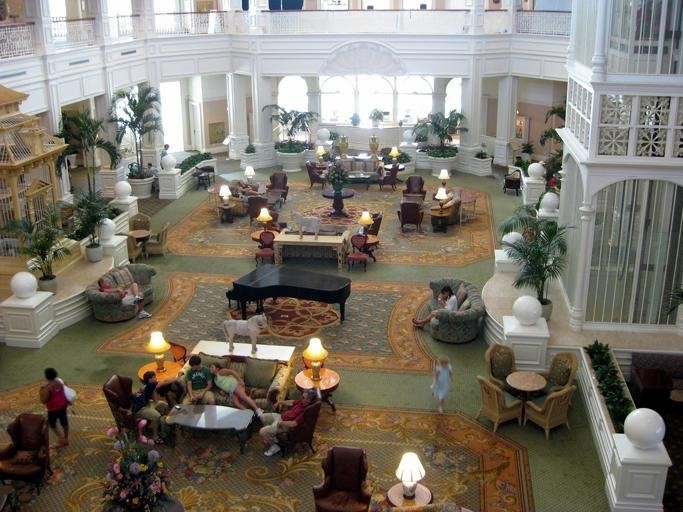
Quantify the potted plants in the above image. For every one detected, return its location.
[105,83,168,200]
[412,107,470,178]
[519,143,536,163]
[261,102,323,173]
[368,108,385,129]
[51,103,120,229]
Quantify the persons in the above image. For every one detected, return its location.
[410,285,458,328]
[39,367,71,448]
[97,277,153,319]
[547,172,560,195]
[258,389,316,458]
[178,354,216,406]
[429,354,454,416]
[144,162,157,177]
[514,156,525,168]
[159,144,169,170]
[209,361,264,419]
[131,370,167,444]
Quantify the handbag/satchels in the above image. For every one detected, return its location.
[63,385,76,402]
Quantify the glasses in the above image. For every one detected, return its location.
[302,396,307,400]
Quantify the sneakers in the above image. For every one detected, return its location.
[138,311,152,319]
[151,434,165,445]
[134,297,144,304]
[264,445,281,457]
[256,408,265,418]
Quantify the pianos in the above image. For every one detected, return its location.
[226,265,350,320]
